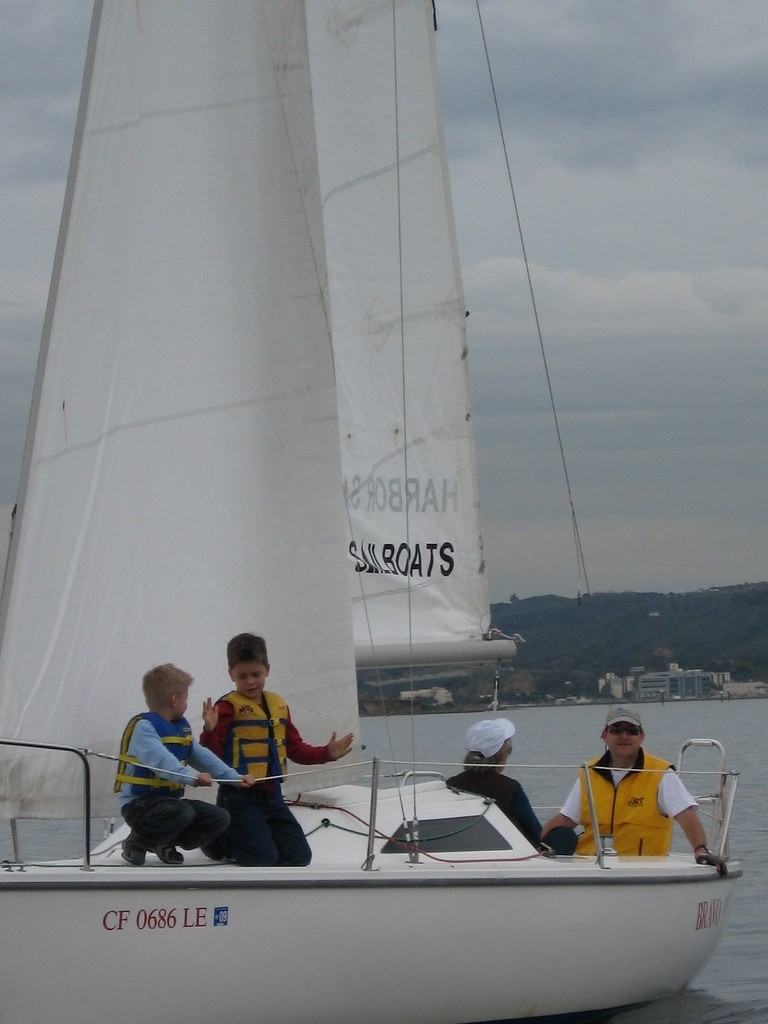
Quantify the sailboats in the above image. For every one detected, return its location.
[1,1,748,1024]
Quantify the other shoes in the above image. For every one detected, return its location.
[201,845,224,860]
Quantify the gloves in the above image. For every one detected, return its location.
[694,845,729,876]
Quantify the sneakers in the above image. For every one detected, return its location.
[148,843,184,864]
[122,835,146,866]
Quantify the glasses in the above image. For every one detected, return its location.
[608,726,641,736]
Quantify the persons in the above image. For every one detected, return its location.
[114,664,257,865]
[540,705,729,878]
[446,717,578,856]
[200,634,354,867]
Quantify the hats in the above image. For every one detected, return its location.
[607,705,642,728]
[466,718,514,761]
